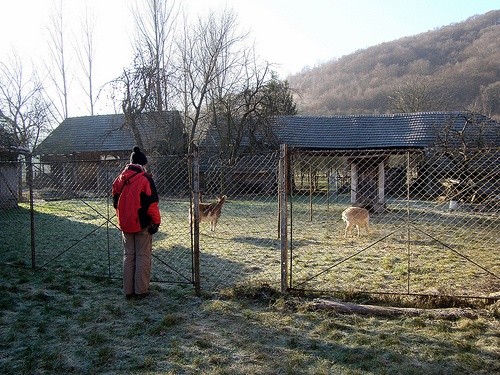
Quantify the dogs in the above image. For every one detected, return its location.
[341,206,370,238]
[189,190,228,232]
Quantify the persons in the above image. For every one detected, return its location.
[112,146,160,297]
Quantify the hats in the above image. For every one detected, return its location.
[129,146,148,165]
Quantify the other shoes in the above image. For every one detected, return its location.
[135,290,150,300]
[126,294,134,300]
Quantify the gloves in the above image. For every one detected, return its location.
[148,224,159,236]
